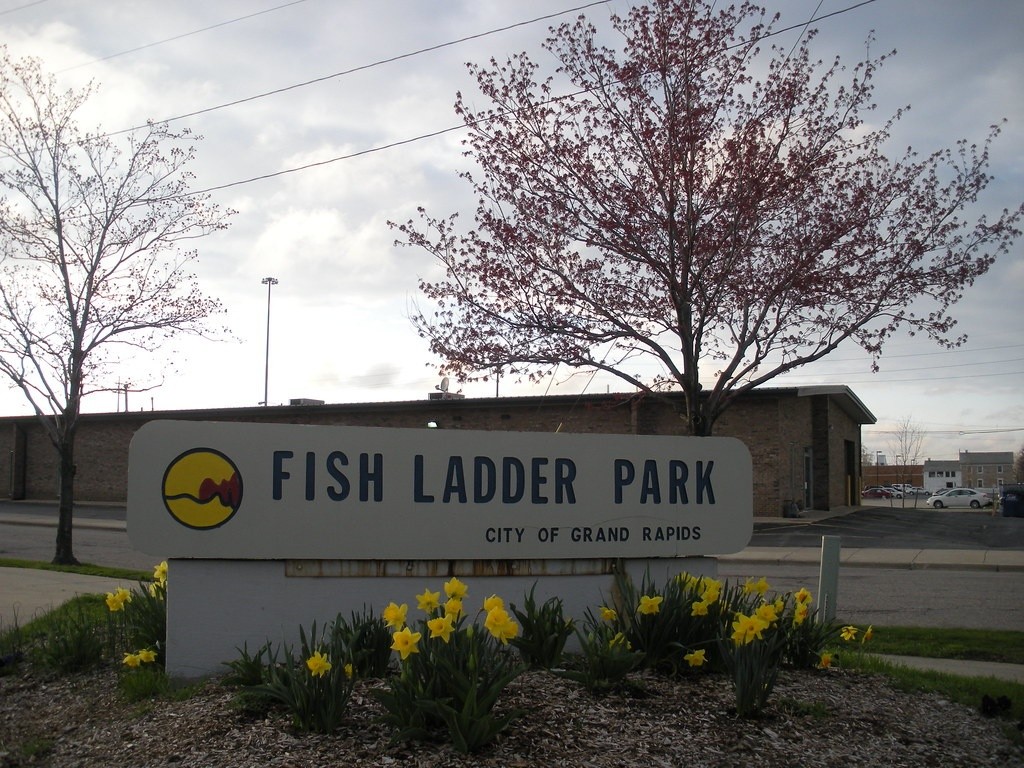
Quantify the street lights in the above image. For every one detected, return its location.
[258,277,279,406]
[896,454,901,490]
[876,450,882,488]
[911,459,915,484]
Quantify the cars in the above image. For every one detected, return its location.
[861,484,912,499]
[926,488,991,510]
[906,487,930,496]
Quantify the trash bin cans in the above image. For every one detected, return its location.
[998,484,1024,518]
